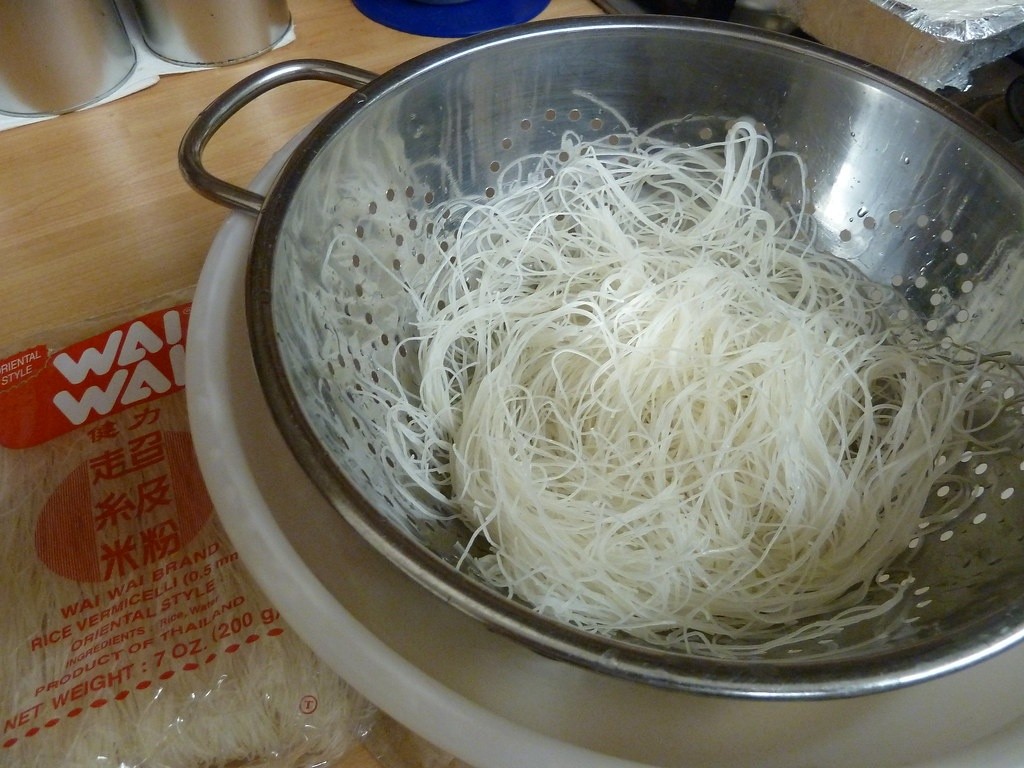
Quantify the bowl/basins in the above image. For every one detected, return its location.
[182,110,1024,768]
[176,15,1024,700]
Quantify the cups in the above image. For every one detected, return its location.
[0,0,137,117]
[130,0,292,66]
[351,0,551,38]
